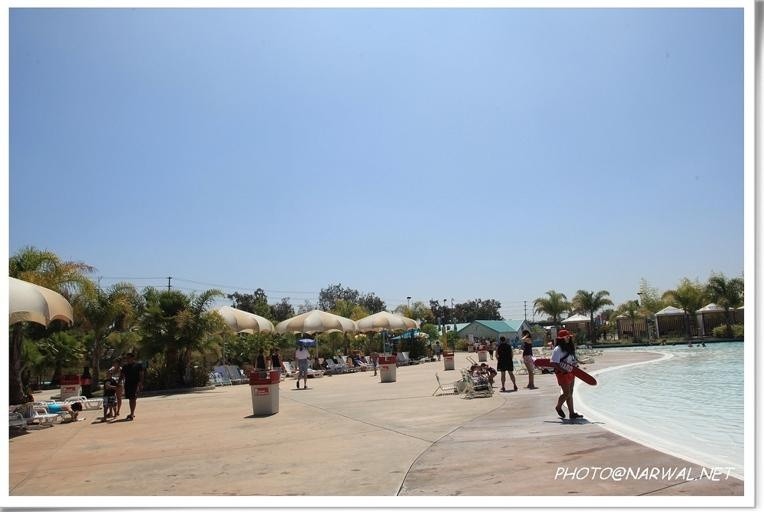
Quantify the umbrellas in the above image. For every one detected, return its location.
[569,412,583,419]
[556,406,565,418]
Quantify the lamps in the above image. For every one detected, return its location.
[431,356,495,399]
[207,355,372,386]
[9,396,103,429]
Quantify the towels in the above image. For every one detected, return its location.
[378,356,396,383]
[442,349,454,370]
[249,368,280,416]
[61,375,80,401]
[477,345,488,362]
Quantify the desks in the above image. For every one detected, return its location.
[407,297,411,309]
[442,298,448,307]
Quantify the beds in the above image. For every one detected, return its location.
[9,277,74,331]
[202,305,274,364]
[354,311,420,361]
[275,310,356,358]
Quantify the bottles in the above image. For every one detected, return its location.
[556,330,572,339]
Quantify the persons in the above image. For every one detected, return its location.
[369,349,380,376]
[101,353,140,420]
[295,342,310,388]
[313,358,321,370]
[550,330,583,419]
[488,341,493,360]
[13,403,82,419]
[522,330,534,388]
[495,336,517,391]
[434,341,441,360]
[270,347,281,377]
[474,341,480,353]
[255,348,267,369]
